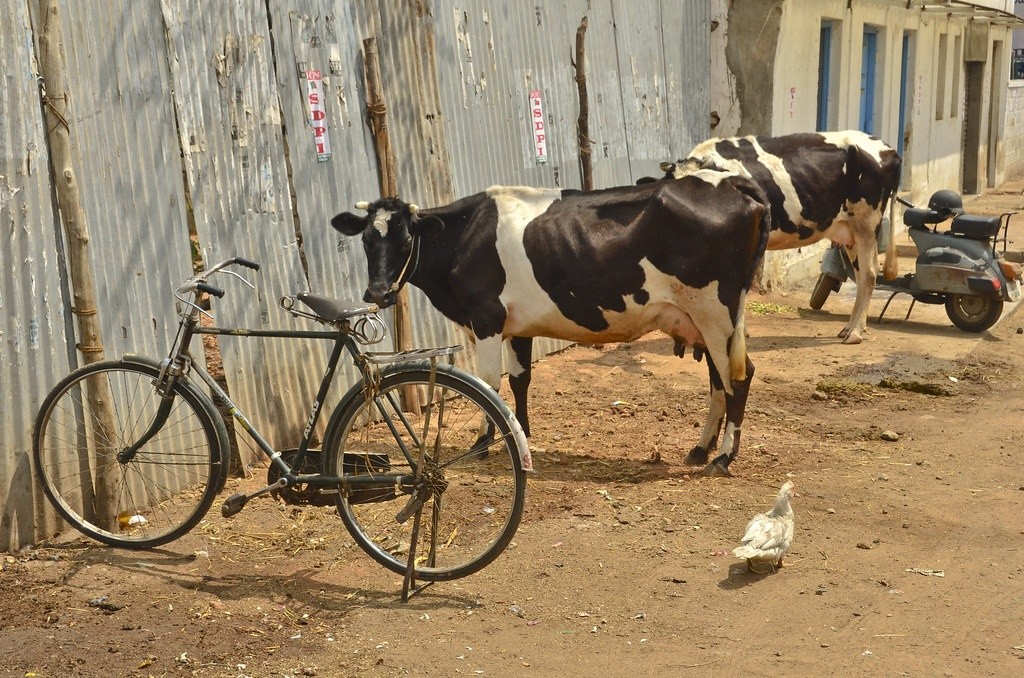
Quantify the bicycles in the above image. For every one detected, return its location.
[29,256,534,605]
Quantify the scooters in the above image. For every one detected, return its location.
[805,188,1018,334]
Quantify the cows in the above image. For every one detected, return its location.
[635,129,904,345]
[327,171,774,480]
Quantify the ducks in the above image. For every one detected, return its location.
[730,480,802,575]
